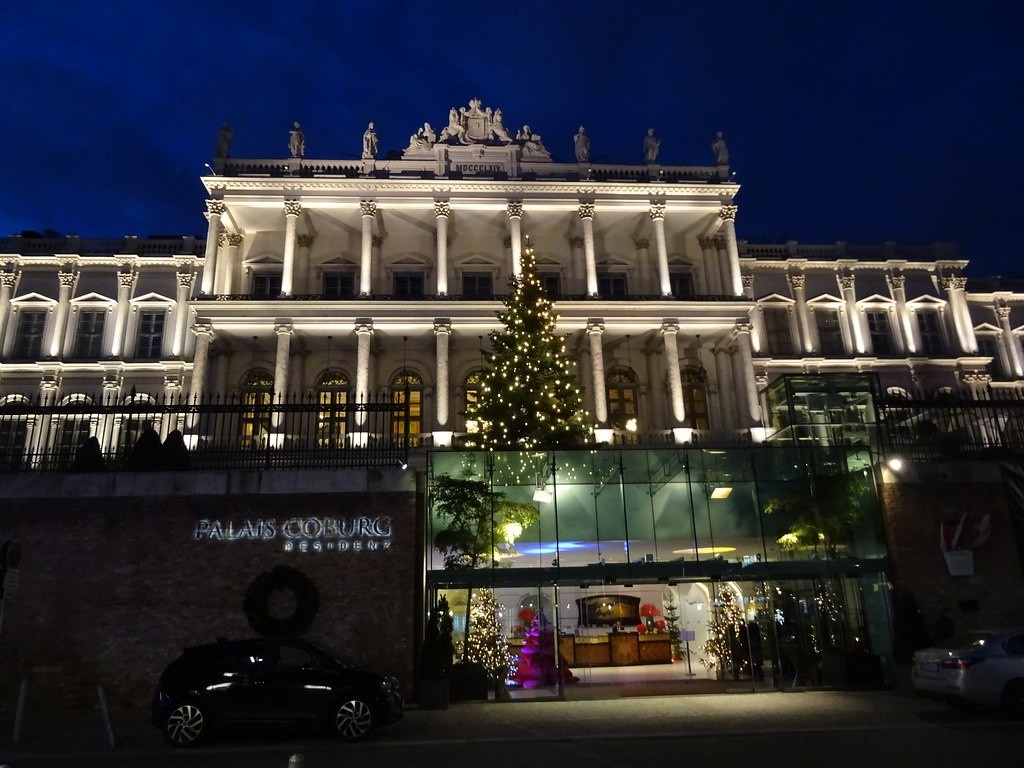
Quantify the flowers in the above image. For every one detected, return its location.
[636,624,647,632]
[512,626,522,634]
[639,602,662,616]
[518,607,536,621]
[654,621,665,629]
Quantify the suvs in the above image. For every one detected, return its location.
[150,637,404,744]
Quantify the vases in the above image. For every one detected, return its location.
[514,632,518,639]
[656,628,663,633]
[524,620,531,637]
[639,630,645,635]
[645,616,655,634]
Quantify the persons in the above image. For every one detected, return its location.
[642,128,662,163]
[359,121,380,158]
[399,108,552,156]
[216,121,233,158]
[288,122,306,157]
[710,130,730,164]
[574,126,592,162]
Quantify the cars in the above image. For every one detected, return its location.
[911,628,1024,712]
